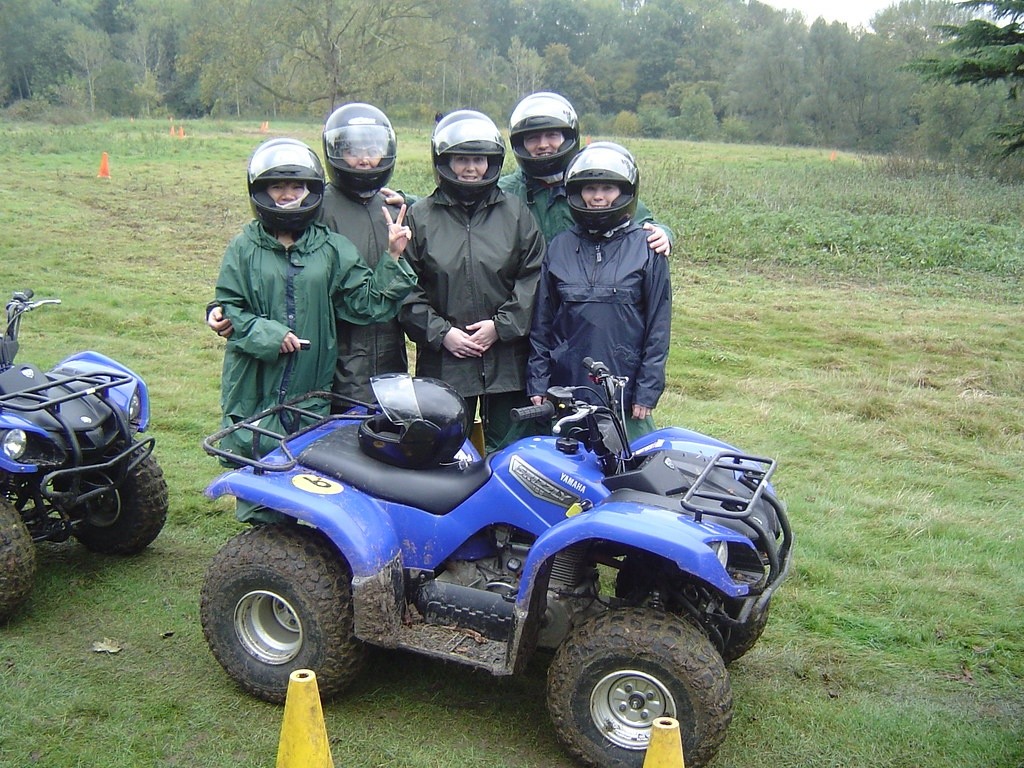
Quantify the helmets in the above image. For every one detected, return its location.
[322,102,397,192]
[247,138,325,229]
[508,92,580,177]
[563,141,640,232]
[431,110,507,200]
[357,372,469,471]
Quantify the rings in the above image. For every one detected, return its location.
[386,223,393,225]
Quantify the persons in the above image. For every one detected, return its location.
[205,102,416,407]
[525,141,672,445]
[399,109,548,454]
[216,140,418,530]
[380,91,674,256]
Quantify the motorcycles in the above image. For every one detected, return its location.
[195,354,796,767]
[1,290,169,619]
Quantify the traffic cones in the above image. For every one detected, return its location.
[170,125,176,138]
[169,117,173,124]
[645,718,687,767]
[96,152,113,180]
[261,120,271,133]
[177,126,185,139]
[275,670,335,768]
[130,117,133,124]
